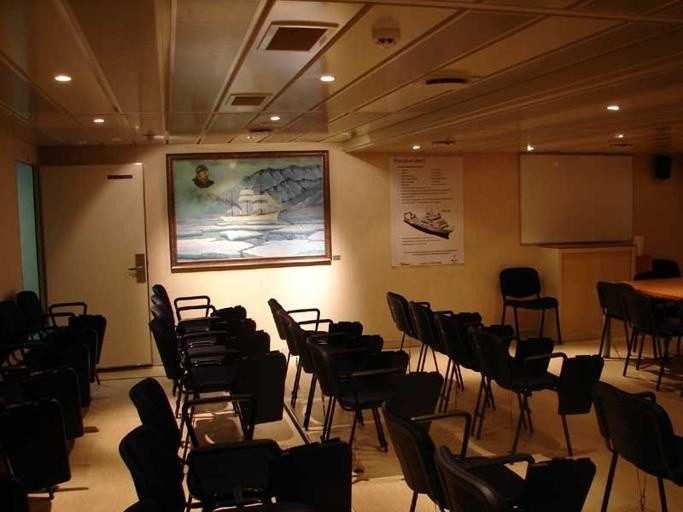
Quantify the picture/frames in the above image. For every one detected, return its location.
[165,150,332,274]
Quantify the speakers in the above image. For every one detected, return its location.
[654,155,671,180]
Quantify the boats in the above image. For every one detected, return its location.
[401,207,455,238]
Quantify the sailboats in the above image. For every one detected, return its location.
[217,181,284,224]
